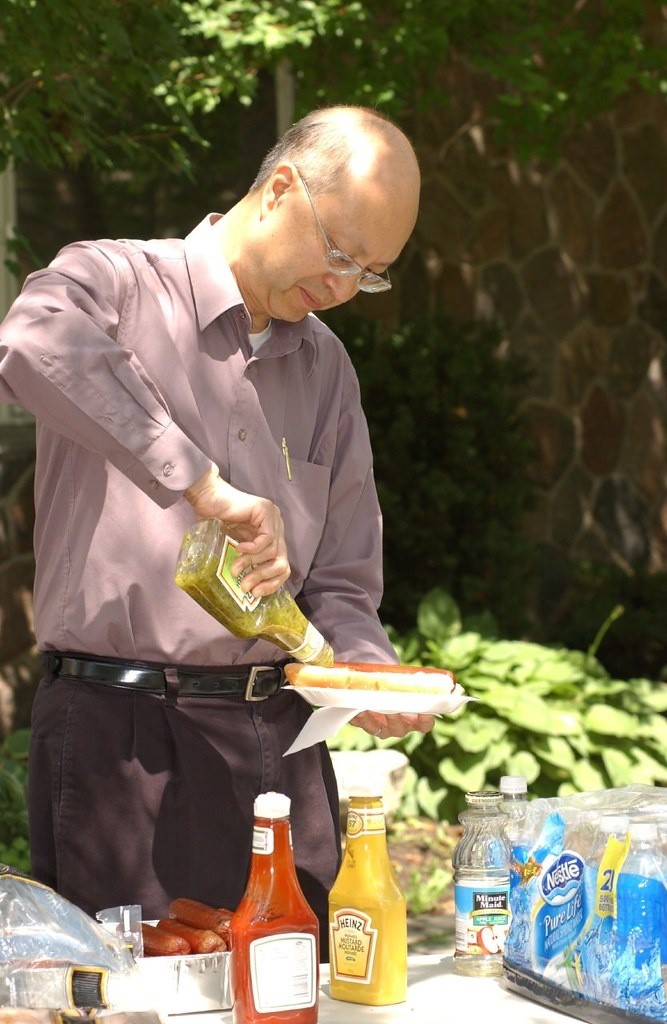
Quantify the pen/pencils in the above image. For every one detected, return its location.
[281,436,296,478]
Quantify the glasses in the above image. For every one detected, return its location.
[294,167,392,293]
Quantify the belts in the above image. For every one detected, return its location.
[42,653,290,702]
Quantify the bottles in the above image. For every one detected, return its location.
[328,796,408,1006]
[499,776,528,843]
[452,791,513,975]
[231,792,319,1024]
[618,823,667,879]
[585,816,628,870]
[174,518,336,666]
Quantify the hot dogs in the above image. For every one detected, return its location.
[284,658,465,694]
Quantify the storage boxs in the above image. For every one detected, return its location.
[12,919,238,1017]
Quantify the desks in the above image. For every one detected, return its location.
[165,954,596,1024]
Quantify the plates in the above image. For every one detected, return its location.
[281,686,480,714]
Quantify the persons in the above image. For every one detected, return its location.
[0,103,435,964]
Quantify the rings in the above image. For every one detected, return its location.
[373,728,381,736]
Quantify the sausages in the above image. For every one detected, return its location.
[113,899,236,955]
